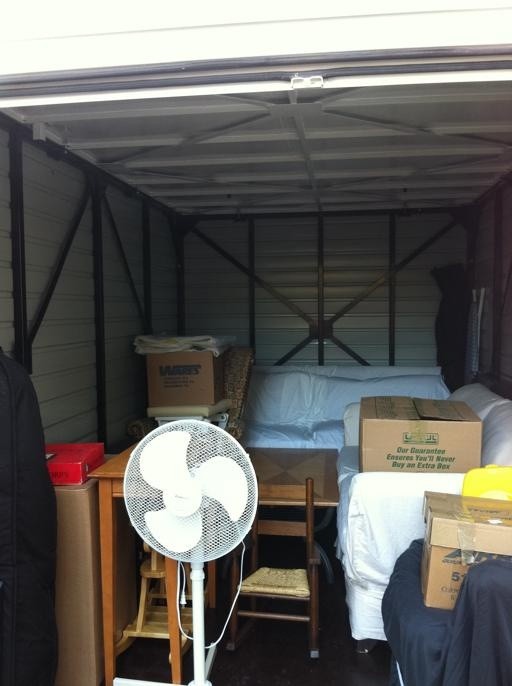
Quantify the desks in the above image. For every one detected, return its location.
[87,442,216,686]
[221,448,339,584]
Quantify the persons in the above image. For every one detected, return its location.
[1,347,61,686]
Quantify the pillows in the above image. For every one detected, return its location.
[248,372,312,424]
[315,374,442,421]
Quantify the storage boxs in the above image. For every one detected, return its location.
[146,351,224,407]
[359,396,483,473]
[421,491,512,611]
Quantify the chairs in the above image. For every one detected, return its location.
[127,347,255,440]
[226,477,321,659]
[382,539,512,686]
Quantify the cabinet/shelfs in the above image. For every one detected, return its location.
[54,454,138,686]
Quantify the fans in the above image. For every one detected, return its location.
[121,418,260,686]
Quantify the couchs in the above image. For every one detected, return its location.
[245,366,452,448]
[333,384,512,654]
